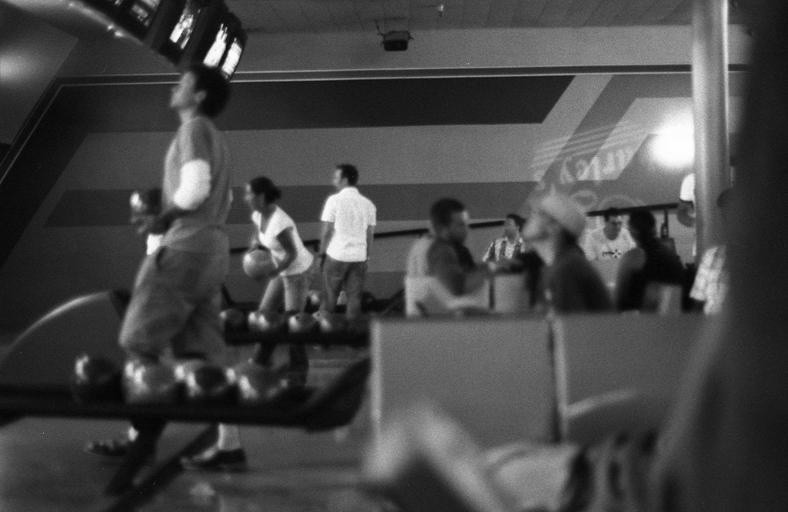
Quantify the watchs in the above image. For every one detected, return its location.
[317,253,326,258]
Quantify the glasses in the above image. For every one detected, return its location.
[609,221,623,225]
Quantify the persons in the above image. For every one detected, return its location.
[404,192,685,318]
[690,187,733,316]
[677,173,697,257]
[245,177,314,379]
[86,65,247,473]
[314,164,377,352]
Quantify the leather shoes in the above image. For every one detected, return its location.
[179,444,248,472]
[85,433,156,465]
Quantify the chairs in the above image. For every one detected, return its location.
[370,316,557,449]
[550,314,695,446]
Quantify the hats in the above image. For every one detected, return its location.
[539,192,586,237]
